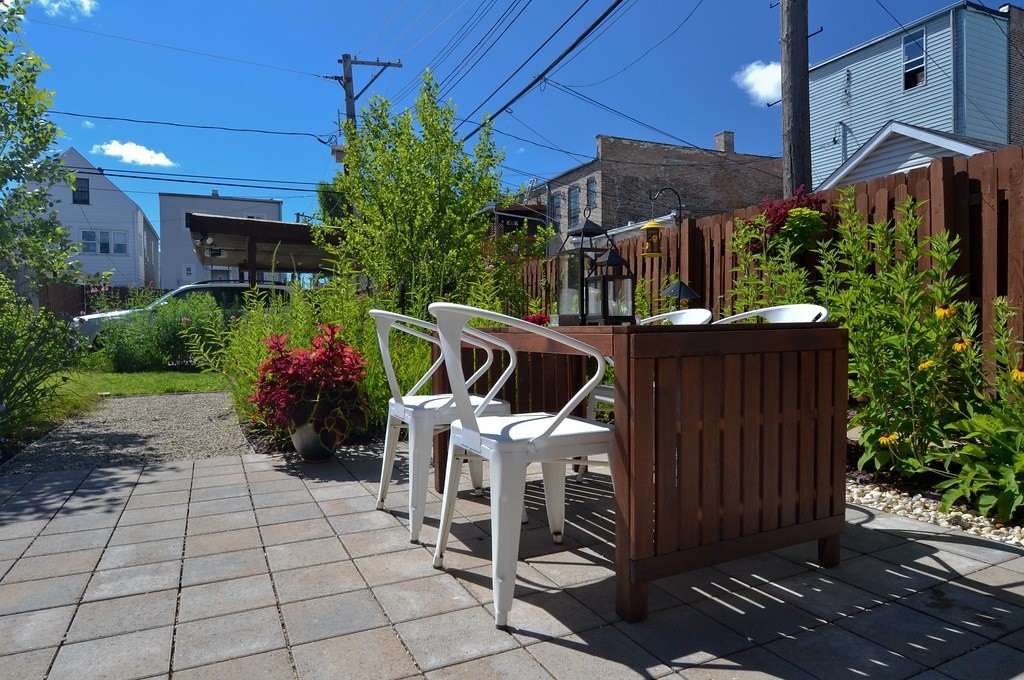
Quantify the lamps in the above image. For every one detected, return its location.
[552,206,638,327]
[193,229,223,258]
[639,199,666,260]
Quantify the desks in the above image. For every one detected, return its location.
[431,323,851,624]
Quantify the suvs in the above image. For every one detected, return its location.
[68,279,320,374]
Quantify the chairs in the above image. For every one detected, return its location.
[576,307,714,488]
[426,301,617,632]
[368,307,530,543]
[711,302,829,323]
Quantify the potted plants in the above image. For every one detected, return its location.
[242,322,371,464]
